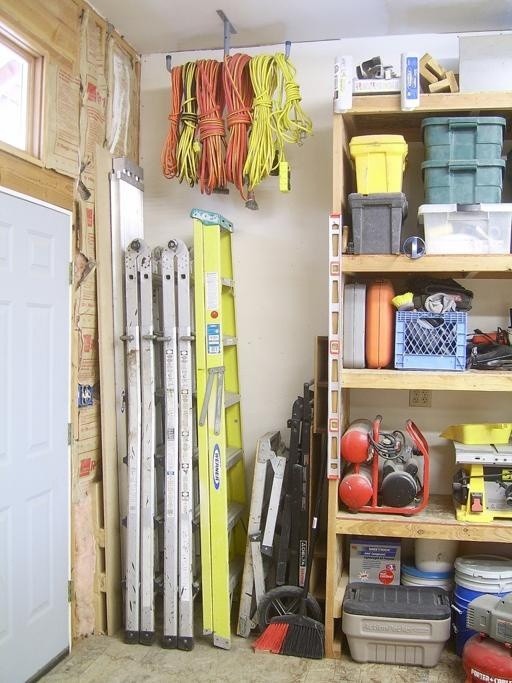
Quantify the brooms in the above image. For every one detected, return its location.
[251,437,327,659]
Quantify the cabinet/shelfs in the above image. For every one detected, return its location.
[323,91,511,661]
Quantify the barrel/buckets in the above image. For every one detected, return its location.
[401,562,454,601]
[452,553,512,658]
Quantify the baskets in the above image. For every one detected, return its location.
[392,308,467,375]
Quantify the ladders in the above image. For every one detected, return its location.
[161,238,202,653]
[121,238,167,645]
[235,429,291,638]
[189,206,249,653]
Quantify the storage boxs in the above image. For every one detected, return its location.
[347,193,408,255]
[365,278,394,369]
[349,537,403,586]
[420,115,507,160]
[416,203,511,254]
[347,133,410,193]
[341,582,453,668]
[344,282,365,368]
[418,160,507,203]
